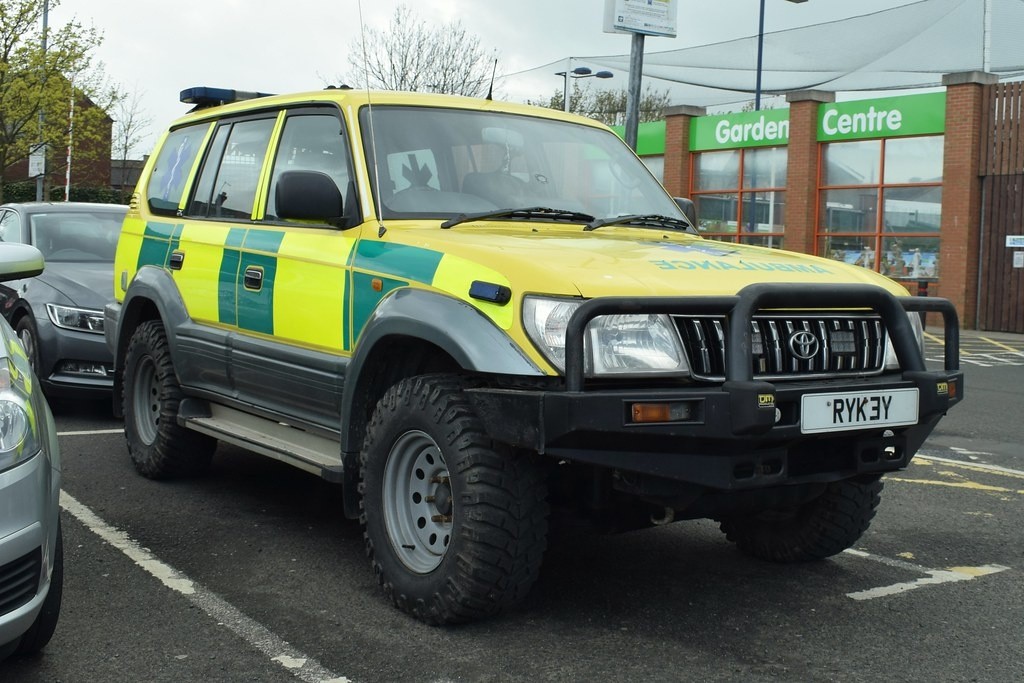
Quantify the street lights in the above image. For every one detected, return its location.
[555,68,613,113]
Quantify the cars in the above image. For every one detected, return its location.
[0,241,62,661]
[0,202,152,407]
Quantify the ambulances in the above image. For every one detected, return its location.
[103,86,964,628]
